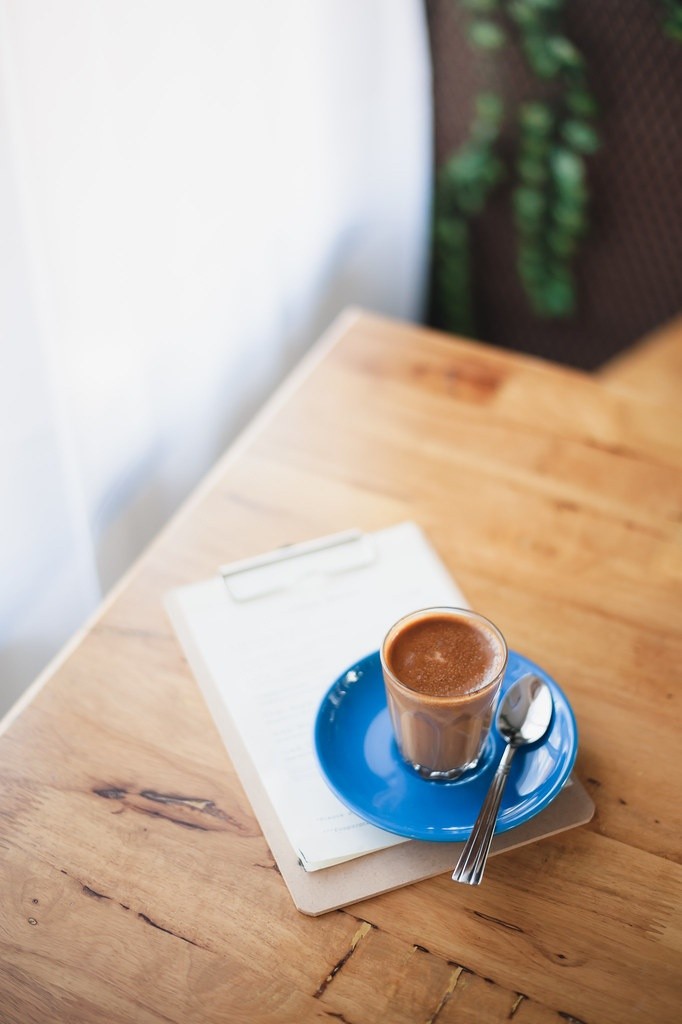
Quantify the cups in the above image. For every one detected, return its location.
[379,606,508,781]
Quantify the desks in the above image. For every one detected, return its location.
[0,309,682,1024]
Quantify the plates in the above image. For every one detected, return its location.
[314,647,579,842]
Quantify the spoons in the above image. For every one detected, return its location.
[451,673,553,886]
